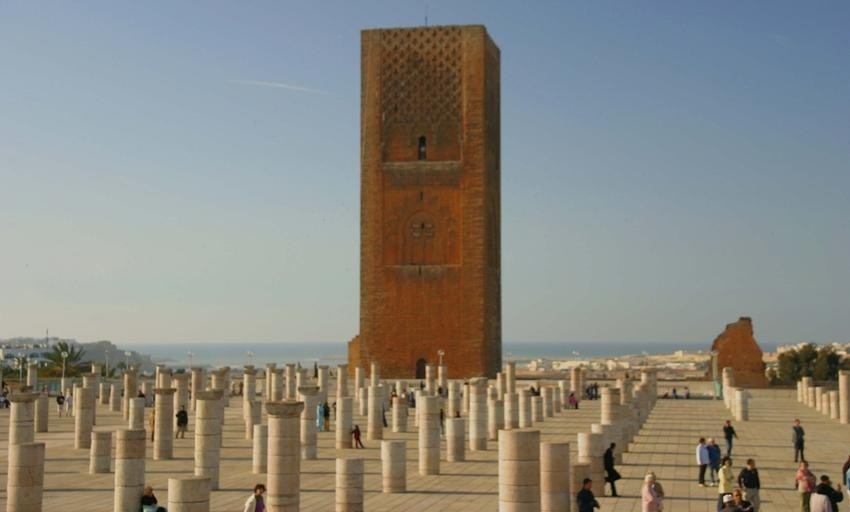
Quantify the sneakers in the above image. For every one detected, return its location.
[698,482,719,487]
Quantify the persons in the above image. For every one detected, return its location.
[576,478,600,512]
[243,483,268,511]
[791,418,807,462]
[0,381,245,441]
[662,392,670,398]
[586,382,599,400]
[641,470,664,511]
[671,387,679,399]
[795,455,850,512]
[696,419,760,511]
[138,485,168,512]
[684,386,691,398]
[316,380,460,449]
[529,384,539,395]
[604,441,622,496]
[567,392,577,408]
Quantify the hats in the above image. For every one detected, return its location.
[723,495,733,503]
